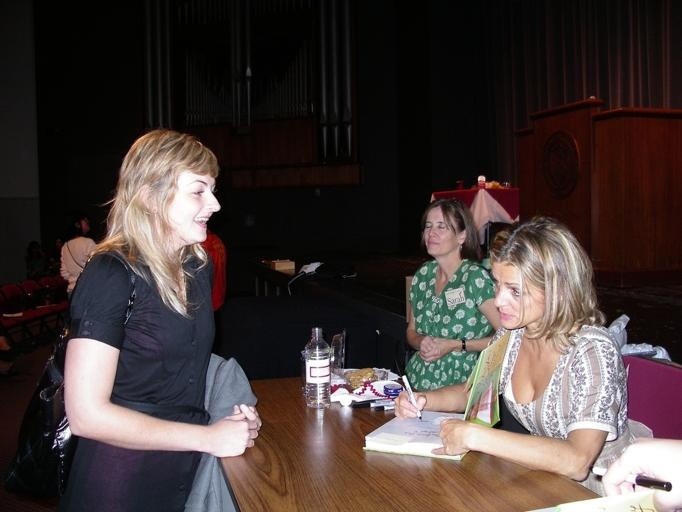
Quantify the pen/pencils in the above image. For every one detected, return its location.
[590,465,671,491]
[352,375,422,421]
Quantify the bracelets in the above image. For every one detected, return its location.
[461,339,466,351]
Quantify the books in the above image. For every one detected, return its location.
[361,328,518,462]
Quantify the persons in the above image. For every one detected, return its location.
[46,235,66,274]
[58,211,97,300]
[0,330,30,380]
[52,127,264,511]
[23,240,46,282]
[400,197,503,416]
[393,213,632,492]
[199,230,228,356]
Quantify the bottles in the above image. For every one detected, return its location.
[303,325,333,408]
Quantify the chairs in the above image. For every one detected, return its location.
[620,352,682,438]
[0,273,73,373]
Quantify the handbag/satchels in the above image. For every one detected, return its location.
[0,355,80,509]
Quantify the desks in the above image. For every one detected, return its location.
[430,182,522,257]
[205,366,604,511]
